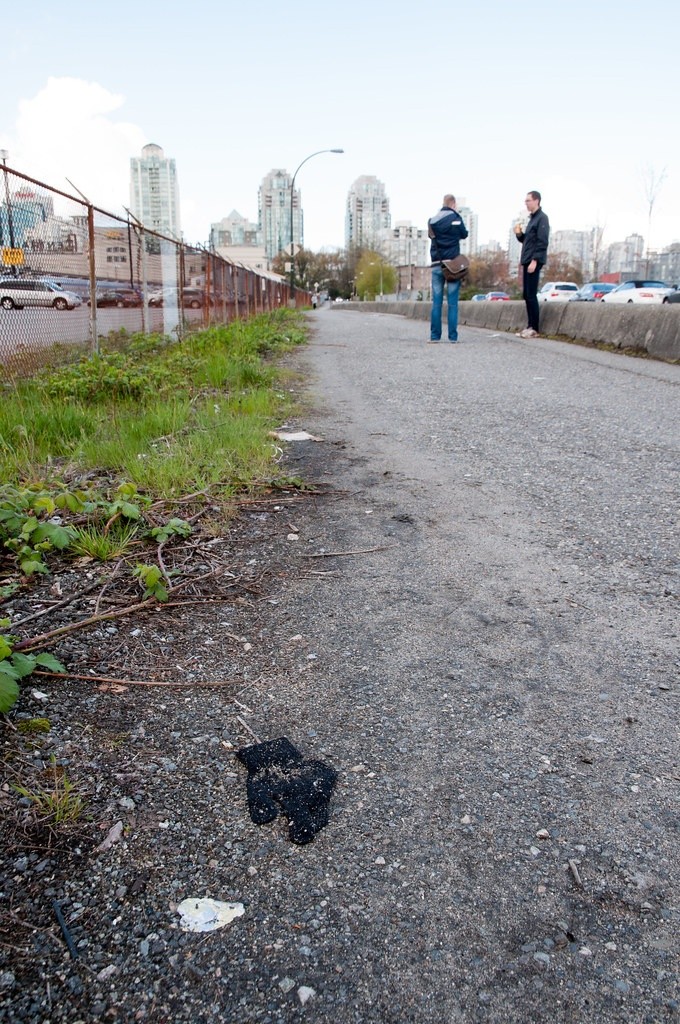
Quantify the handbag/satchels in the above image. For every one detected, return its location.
[440,254,470,281]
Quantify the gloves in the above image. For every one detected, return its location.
[235,737,337,846]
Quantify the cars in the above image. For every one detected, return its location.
[481,292,512,300]
[662,283,680,303]
[599,279,674,304]
[568,283,617,302]
[471,294,485,301]
[87,288,249,308]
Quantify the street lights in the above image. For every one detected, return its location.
[1,148,17,278]
[289,148,344,299]
[370,262,383,302]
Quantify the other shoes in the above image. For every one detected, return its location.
[516,328,540,339]
[426,338,440,344]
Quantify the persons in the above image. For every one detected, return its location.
[514,191,549,338]
[311,294,317,310]
[428,194,468,343]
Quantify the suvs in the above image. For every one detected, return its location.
[537,282,578,301]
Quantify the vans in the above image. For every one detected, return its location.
[0,278,84,310]
